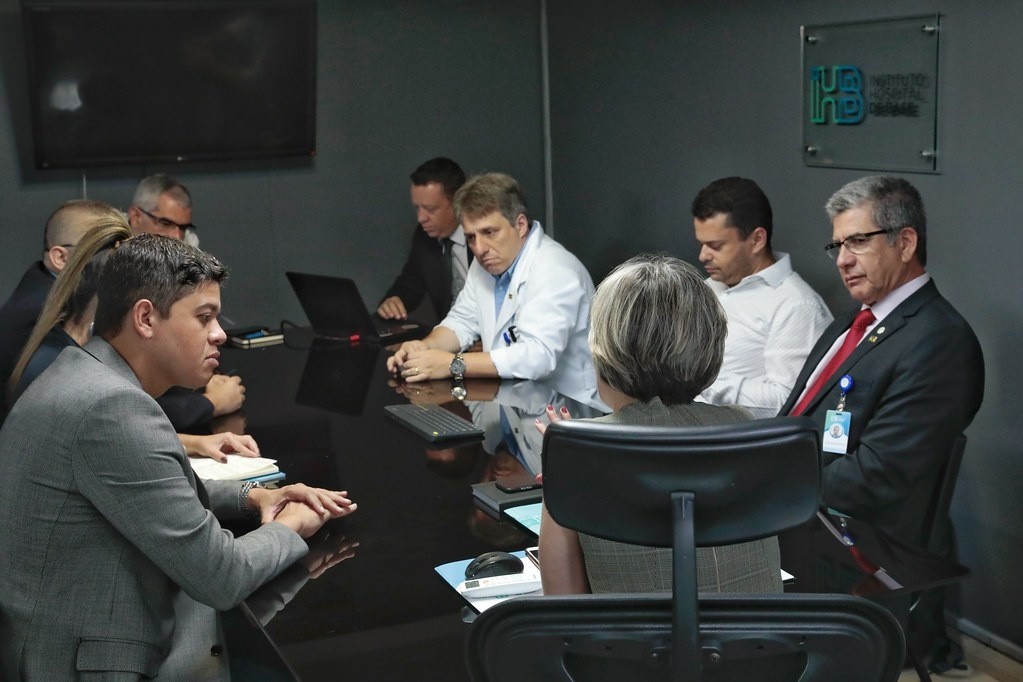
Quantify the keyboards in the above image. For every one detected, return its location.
[384,402,486,442]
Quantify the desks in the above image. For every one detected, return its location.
[176,307,974,682]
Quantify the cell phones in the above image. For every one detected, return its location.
[526,546,540,570]
[494,476,543,493]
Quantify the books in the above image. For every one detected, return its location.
[244,472,286,484]
[230,332,284,344]
[186,454,280,481]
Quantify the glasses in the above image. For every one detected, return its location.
[825,225,911,260]
[137,206,195,238]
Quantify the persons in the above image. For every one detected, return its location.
[831,426,840,437]
[772,174,984,632]
[687,175,833,416]
[377,158,476,322]
[389,379,607,547]
[534,253,782,594]
[0,171,357,682]
[386,172,612,414]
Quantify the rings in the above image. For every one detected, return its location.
[416,368,419,374]
[417,390,420,394]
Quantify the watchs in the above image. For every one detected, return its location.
[450,381,466,401]
[450,352,465,379]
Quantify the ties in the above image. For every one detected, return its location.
[792,307,875,415]
[441,237,455,304]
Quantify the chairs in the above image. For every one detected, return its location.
[819,432,973,682]
[464,413,907,682]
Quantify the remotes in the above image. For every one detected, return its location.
[460,571,544,598]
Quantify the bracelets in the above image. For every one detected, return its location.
[240,481,261,513]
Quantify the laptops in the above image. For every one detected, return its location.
[286,271,425,343]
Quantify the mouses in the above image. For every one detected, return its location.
[465,551,524,580]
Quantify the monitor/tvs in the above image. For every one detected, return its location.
[20,0,316,168]
